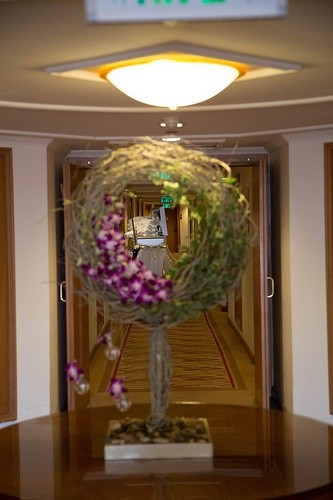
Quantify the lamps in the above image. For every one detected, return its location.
[43,39,312,111]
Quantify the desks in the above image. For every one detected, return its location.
[0,403,333,500]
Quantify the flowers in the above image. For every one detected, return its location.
[59,194,174,411]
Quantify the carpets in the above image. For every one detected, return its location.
[94,310,248,392]
